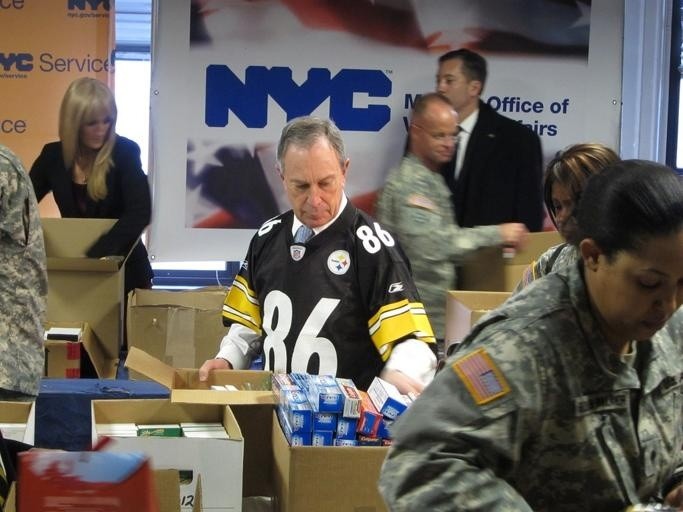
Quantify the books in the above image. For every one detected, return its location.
[94,421,230,440]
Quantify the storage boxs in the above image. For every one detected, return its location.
[126,345,281,497]
[90,398,243,512]
[446,289,516,361]
[1,402,35,447]
[272,374,431,512]
[128,285,251,371]
[456,230,567,296]
[41,319,119,379]
[38,217,148,356]
[1,470,202,512]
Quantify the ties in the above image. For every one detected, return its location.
[294,226,313,246]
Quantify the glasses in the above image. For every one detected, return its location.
[420,127,461,143]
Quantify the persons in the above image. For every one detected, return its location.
[0,142,50,401]
[403,48,544,233]
[197,115,439,398]
[513,142,682,291]
[374,92,528,350]
[28,76,154,348]
[373,157,682,512]
